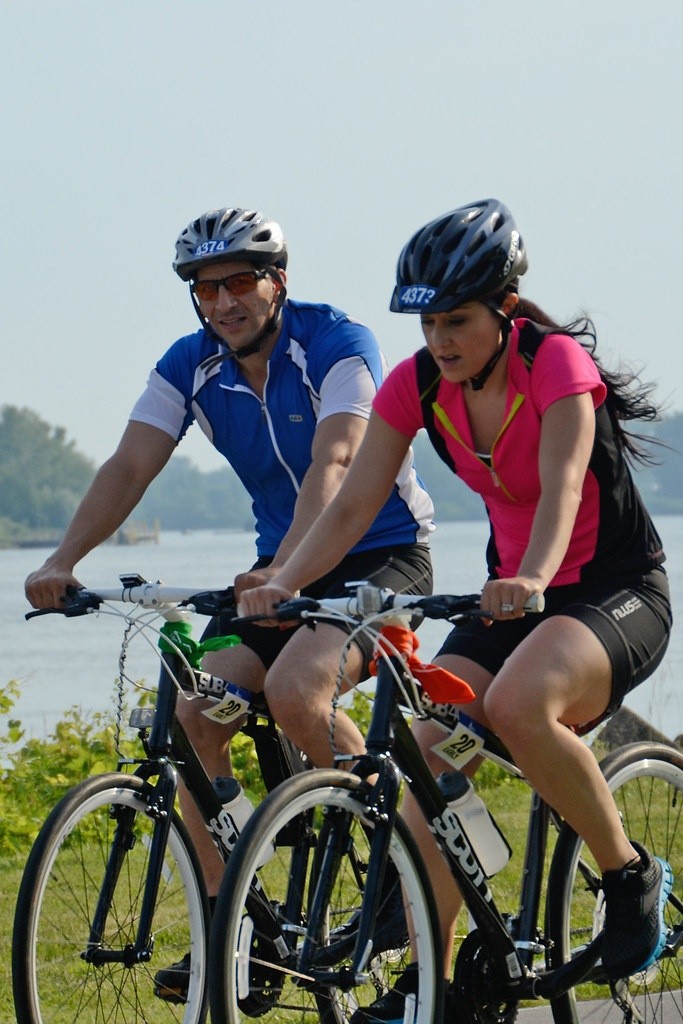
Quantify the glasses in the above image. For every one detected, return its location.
[190,270,267,299]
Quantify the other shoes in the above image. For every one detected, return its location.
[154,954,192,991]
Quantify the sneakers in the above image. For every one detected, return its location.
[601,841,674,977]
[350,962,450,1024]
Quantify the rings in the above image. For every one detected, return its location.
[501,604,515,611]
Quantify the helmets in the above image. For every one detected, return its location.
[172,207,288,280]
[390,198,527,314]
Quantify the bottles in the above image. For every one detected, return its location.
[211,774,275,872]
[436,769,510,877]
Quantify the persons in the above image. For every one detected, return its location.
[241,196,675,1024]
[25,206,438,1006]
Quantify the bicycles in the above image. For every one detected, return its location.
[6,575,557,1024]
[201,585,683,1024]
